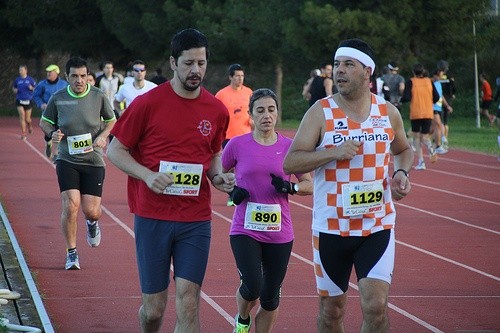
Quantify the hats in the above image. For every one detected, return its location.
[45,64,60,74]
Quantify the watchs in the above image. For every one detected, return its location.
[47,131,55,140]
[290,181,298,196]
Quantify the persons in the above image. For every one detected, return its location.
[213,64,254,206]
[221,90,314,333]
[12,64,37,141]
[283,40,414,333]
[370,58,455,169]
[477,71,496,127]
[106,28,235,333]
[32,65,69,164]
[302,62,333,108]
[87,60,168,146]
[39,57,117,270]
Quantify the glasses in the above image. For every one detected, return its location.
[133,68,145,72]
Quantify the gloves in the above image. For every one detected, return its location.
[228,185,250,206]
[270,173,299,196]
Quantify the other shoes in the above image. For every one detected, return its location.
[29,129,32,133]
[415,161,426,170]
[442,136,448,143]
[429,149,435,157]
[435,146,449,154]
[22,136,26,140]
[489,114,496,124]
[227,197,234,206]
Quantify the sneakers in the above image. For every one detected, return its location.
[86,219,101,247]
[233,313,252,333]
[46,140,52,158]
[65,247,81,270]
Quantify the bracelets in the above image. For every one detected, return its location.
[211,174,218,188]
[392,168,409,179]
